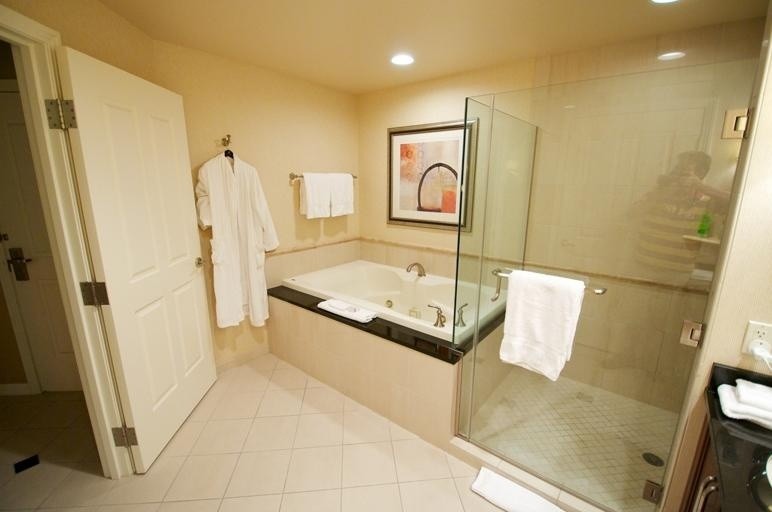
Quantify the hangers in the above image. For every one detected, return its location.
[225,139,233,159]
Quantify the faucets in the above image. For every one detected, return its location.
[407,262,426,276]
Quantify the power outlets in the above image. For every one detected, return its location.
[740,319,772,358]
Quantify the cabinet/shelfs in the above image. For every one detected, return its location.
[679,412,720,512]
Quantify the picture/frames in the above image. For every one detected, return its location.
[384,117,480,234]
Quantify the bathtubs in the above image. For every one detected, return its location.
[282,260,508,345]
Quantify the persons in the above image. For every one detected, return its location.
[598,149,733,386]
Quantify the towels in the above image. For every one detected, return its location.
[316,298,379,325]
[298,172,354,218]
[498,269,585,382]
[717,378,772,430]
[468,467,567,511]
